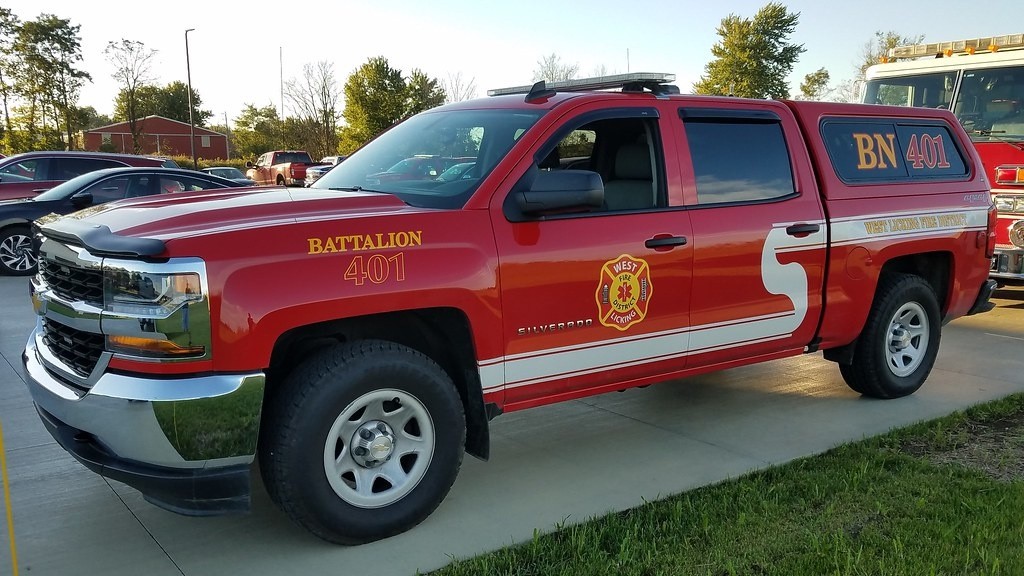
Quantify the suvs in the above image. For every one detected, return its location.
[363,154,478,186]
[303,154,346,188]
[0,150,194,201]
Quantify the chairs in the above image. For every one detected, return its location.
[602,142,654,211]
[539,145,561,171]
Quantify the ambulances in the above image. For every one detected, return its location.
[20,71,997,548]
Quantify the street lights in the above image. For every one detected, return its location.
[184,28,198,170]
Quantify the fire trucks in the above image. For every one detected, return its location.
[859,33,1024,285]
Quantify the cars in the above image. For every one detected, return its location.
[558,155,589,173]
[199,167,258,186]
[0,166,253,276]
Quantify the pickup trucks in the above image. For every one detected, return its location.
[246,150,333,188]
